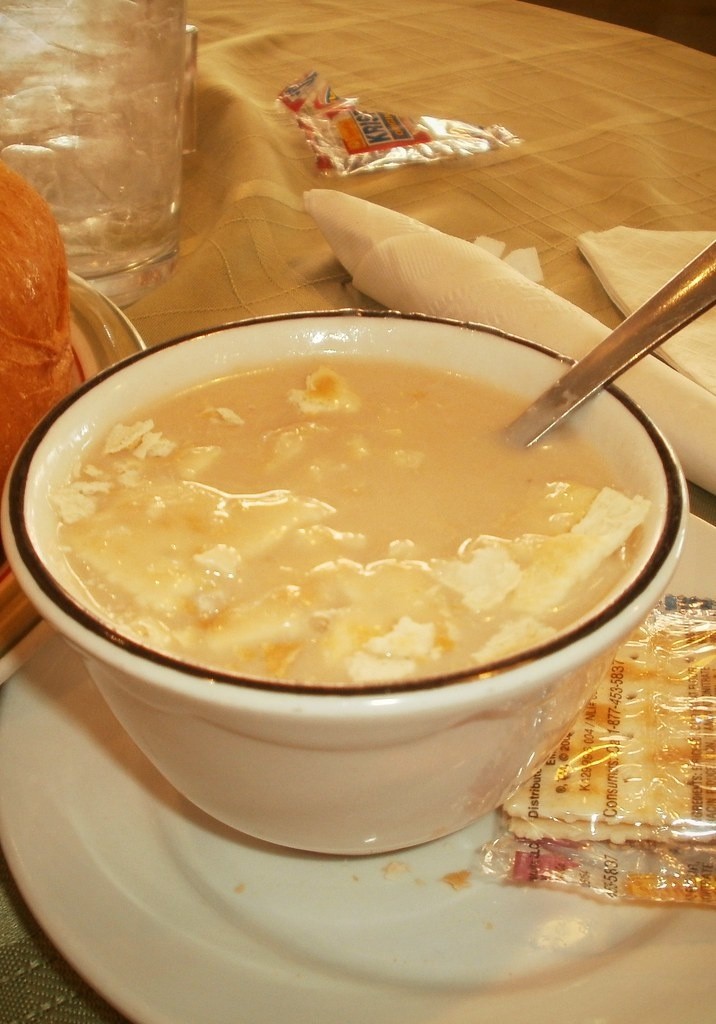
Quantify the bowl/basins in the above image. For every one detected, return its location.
[2,307,692,857]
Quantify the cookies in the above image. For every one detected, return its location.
[504,613,716,844]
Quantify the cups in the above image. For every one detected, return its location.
[2,2,196,311]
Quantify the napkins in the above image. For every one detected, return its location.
[575,224,716,395]
[303,187,716,495]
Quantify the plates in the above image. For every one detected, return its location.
[2,268,149,686]
[0,512,716,1024]
